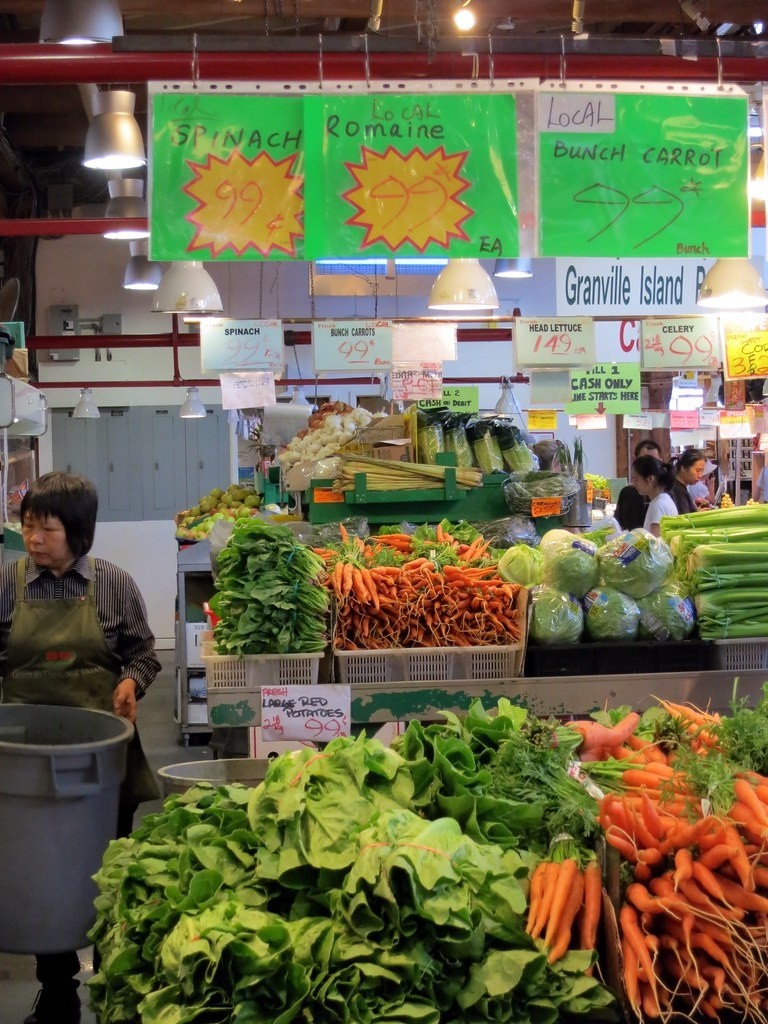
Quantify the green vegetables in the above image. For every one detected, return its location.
[414,409,582,500]
[208,517,328,661]
[84,694,616,1024]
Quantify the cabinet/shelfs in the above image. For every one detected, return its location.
[175,544,216,747]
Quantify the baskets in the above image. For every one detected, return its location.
[334,636,768,682]
[202,631,325,689]
[356,425,405,443]
[502,477,580,516]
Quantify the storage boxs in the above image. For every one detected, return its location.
[202,602,767,689]
[187,703,208,725]
[283,457,328,492]
[186,622,209,669]
[371,442,415,463]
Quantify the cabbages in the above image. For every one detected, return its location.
[497,528,696,643]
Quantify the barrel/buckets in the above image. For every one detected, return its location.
[159,758,273,794]
[1,703,135,953]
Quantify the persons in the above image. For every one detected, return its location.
[254,448,275,498]
[753,466,768,503]
[613,439,676,533]
[0,471,163,1024]
[670,449,708,515]
[632,455,679,538]
[686,458,718,505]
[529,439,580,538]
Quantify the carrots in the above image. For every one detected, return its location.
[307,520,524,649]
[522,698,768,1024]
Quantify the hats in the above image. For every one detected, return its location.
[701,459,718,475]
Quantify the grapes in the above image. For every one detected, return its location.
[583,473,607,490]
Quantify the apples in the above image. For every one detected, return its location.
[746,498,760,505]
[176,483,261,541]
[721,494,734,508]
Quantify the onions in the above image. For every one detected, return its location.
[276,399,389,468]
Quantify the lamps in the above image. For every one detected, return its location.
[123,240,165,291]
[179,385,207,419]
[697,259,768,309]
[492,259,533,278]
[81,83,145,169]
[72,388,101,419]
[494,380,521,414]
[151,261,224,314]
[290,385,310,405]
[426,258,500,310]
[104,179,150,240]
[40,0,124,45]
